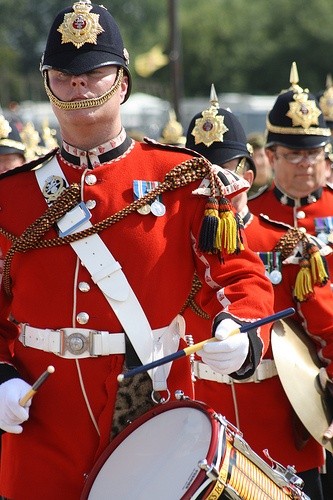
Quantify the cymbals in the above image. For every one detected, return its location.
[266,315,333,458]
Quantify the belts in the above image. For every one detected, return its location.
[16,323,169,359]
[193,359,278,383]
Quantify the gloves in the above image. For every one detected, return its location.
[195,318,249,374]
[0,378,33,433]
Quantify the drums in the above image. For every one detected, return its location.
[79,396,312,500]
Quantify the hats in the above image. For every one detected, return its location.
[0,107,26,161]
[38,1,131,104]
[18,119,60,162]
[185,83,256,182]
[264,62,331,149]
[316,74,333,129]
[157,110,186,149]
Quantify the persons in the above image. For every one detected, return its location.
[1,0,274,497]
[0,112,59,175]
[247,61,333,250]
[246,129,274,196]
[186,81,333,500]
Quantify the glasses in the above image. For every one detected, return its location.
[277,146,323,164]
